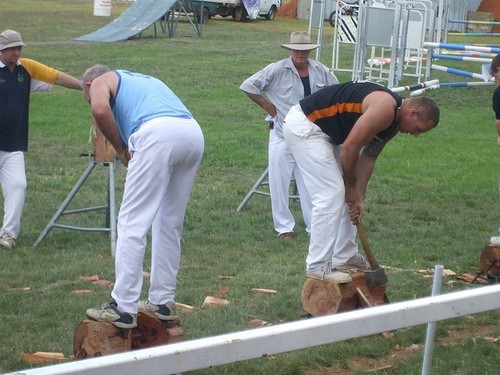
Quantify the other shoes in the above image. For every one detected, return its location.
[280,232,294,240]
[490,236,499,246]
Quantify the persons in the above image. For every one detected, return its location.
[0,30,83,251]
[283,80,440,283]
[490,54,500,146]
[240,31,339,240]
[83,65,204,329]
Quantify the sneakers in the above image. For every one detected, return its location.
[139,299,176,321]
[306,262,353,283]
[345,253,370,269]
[0,233,16,249]
[85,298,138,329]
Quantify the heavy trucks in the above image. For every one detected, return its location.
[157,0,283,25]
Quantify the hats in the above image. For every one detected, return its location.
[280,30,320,52]
[0,30,26,51]
